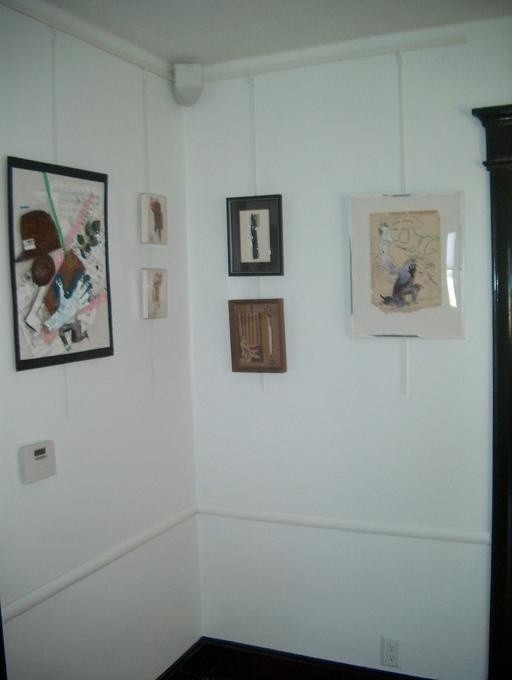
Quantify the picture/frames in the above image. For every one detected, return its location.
[227,299,288,374]
[347,188,464,340]
[226,193,283,277]
[139,192,170,246]
[8,155,113,372]
[142,268,169,321]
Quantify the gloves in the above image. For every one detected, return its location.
[45,270,93,330]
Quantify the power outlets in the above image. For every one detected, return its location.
[380,636,403,668]
